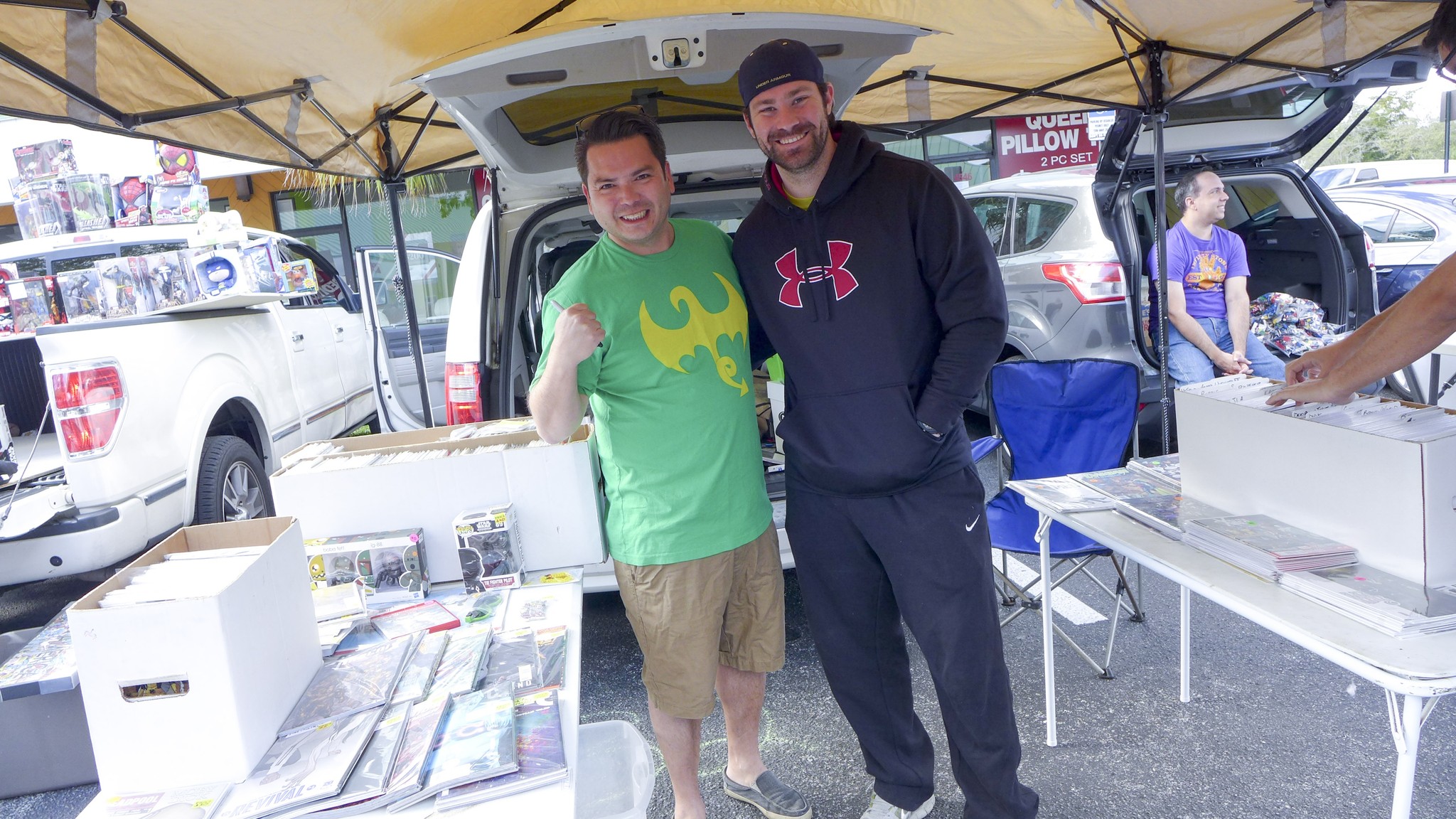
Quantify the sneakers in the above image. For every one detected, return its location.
[860,789,935,819]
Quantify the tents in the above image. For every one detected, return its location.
[1,0,1442,459]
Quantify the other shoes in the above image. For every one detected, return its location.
[722,765,812,819]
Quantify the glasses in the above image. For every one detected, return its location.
[1436,46,1456,84]
[575,105,647,146]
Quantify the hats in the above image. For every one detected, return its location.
[738,38,824,108]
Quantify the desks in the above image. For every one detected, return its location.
[77,568,585,819]
[1024,493,1456,819]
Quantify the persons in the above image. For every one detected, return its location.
[63,252,190,324]
[527,98,817,817]
[715,35,1045,818]
[1147,167,1289,402]
[1268,1,1456,410]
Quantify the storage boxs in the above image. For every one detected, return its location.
[0,601,80,700]
[451,502,528,594]
[0,626,99,799]
[1174,374,1456,588]
[280,415,534,468]
[302,537,327,591]
[0,139,318,337]
[65,517,323,793]
[269,423,608,582]
[321,528,432,605]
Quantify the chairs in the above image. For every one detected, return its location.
[970,358,1147,678]
